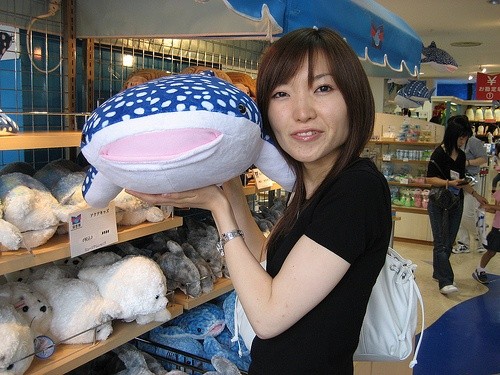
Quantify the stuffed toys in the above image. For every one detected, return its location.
[0,70,297,375]
[394,41,459,110]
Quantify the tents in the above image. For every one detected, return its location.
[223,0,421,79]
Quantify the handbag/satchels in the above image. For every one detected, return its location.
[229,248,419,361]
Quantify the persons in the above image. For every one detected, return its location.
[401,108,411,118]
[425,115,500,294]
[125,27,392,375]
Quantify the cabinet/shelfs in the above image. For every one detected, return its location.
[361,112,446,244]
[1,131,284,375]
[431,97,500,169]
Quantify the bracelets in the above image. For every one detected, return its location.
[215,229,245,256]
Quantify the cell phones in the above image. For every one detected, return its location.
[456,178,471,186]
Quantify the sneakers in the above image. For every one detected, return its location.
[453,245,470,253]
[473,270,490,283]
[478,246,486,252]
[439,285,457,294]
